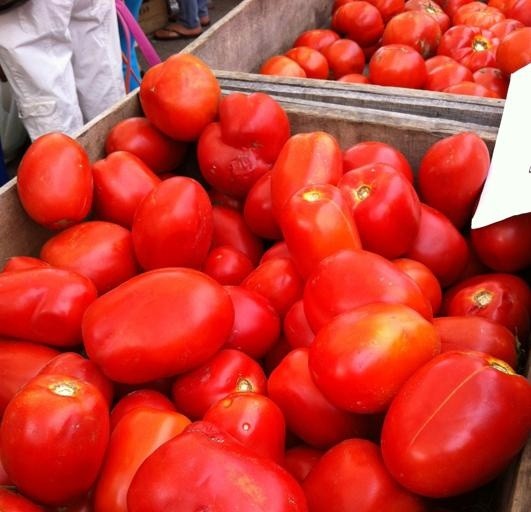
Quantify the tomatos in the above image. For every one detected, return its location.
[258,0,531,99]
[0,51,531,512]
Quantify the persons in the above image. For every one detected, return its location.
[0,0,127,144]
[119,0,143,92]
[153,0,211,41]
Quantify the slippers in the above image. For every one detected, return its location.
[152,23,202,40]
[200,16,211,27]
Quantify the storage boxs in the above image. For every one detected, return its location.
[1,81,530,512]
[175,0,531,124]
